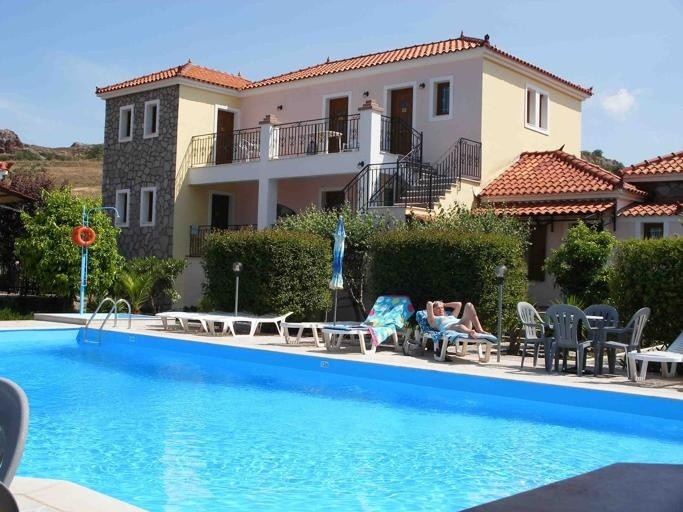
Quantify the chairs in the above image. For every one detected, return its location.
[233,127,358,163]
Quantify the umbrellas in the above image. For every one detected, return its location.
[327,206,346,325]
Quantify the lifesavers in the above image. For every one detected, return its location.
[73,226,95,246]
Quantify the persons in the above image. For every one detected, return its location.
[425,300,489,340]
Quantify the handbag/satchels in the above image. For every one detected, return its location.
[404,339,424,356]
